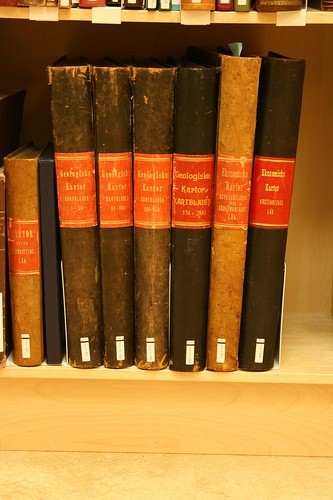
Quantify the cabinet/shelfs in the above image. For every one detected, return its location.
[1,1,333,498]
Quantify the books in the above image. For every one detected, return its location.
[0,0,333,374]
